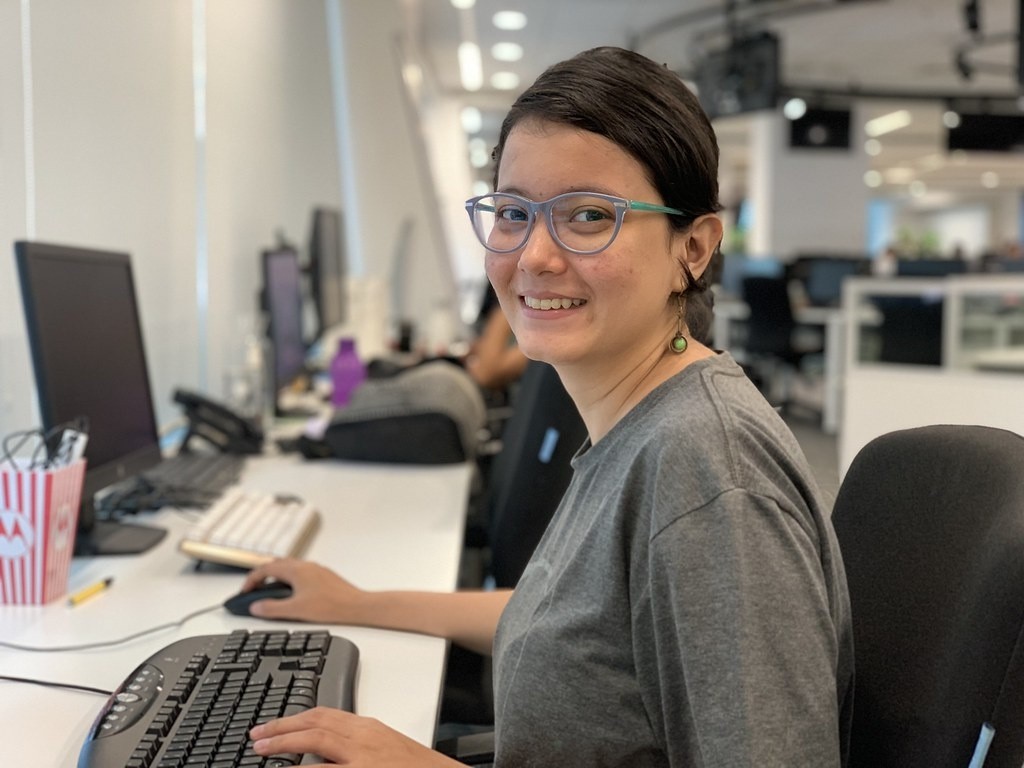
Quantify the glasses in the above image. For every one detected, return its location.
[465,192,686,254]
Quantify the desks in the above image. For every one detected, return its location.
[0,390,475,768]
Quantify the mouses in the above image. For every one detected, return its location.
[225,580,293,616]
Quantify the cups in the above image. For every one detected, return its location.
[0,459,87,606]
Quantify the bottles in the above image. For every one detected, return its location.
[334,341,363,407]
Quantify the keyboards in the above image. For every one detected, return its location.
[78,627,361,768]
[143,451,243,506]
[180,487,321,570]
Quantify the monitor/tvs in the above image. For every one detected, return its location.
[791,108,851,148]
[795,255,869,309]
[12,242,168,554]
[898,259,967,280]
[310,209,345,332]
[263,248,316,420]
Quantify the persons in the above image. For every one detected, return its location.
[472,280,531,389]
[240,48,856,768]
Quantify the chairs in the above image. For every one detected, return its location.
[739,272,829,418]
[431,426,1024,768]
[439,358,588,728]
[869,295,946,368]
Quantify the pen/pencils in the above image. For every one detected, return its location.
[68,577,115,608]
